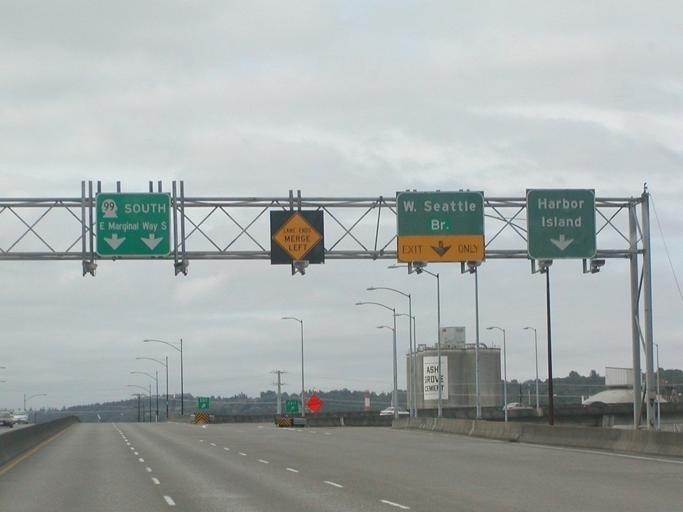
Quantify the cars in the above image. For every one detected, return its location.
[503,402,532,410]
[380,406,410,418]
[0,410,15,428]
[13,410,29,424]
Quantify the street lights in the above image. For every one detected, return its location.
[522,326,540,408]
[122,337,185,422]
[386,264,444,417]
[486,322,509,422]
[281,315,305,418]
[23,389,48,409]
[356,284,418,417]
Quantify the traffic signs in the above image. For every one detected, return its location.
[526,188,597,259]
[93,194,170,257]
